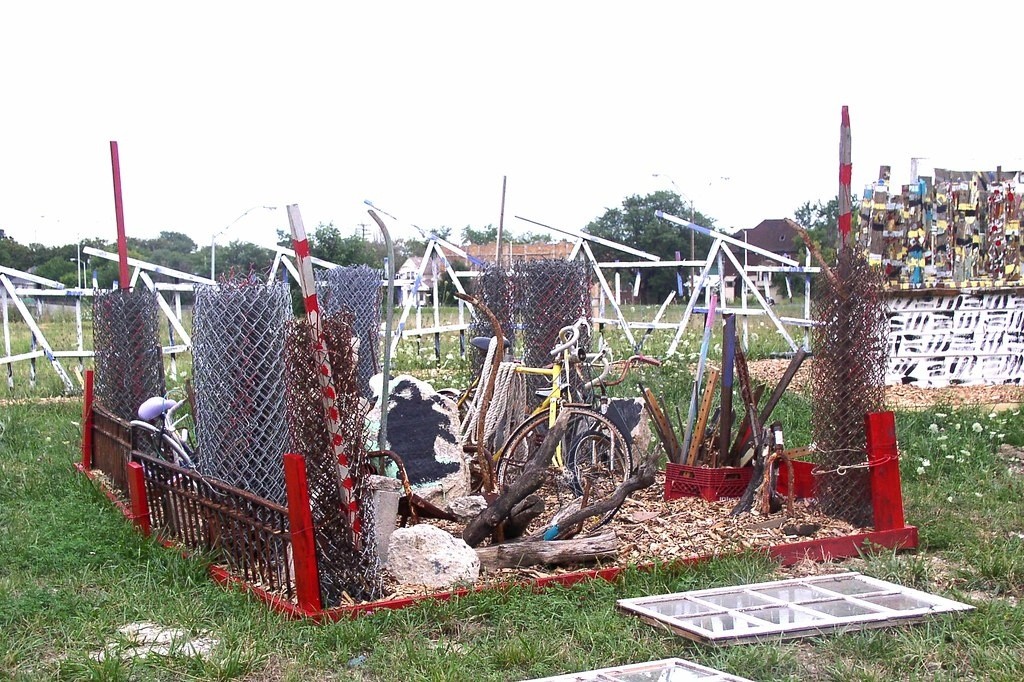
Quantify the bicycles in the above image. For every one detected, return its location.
[442,321,663,537]
[131,387,200,473]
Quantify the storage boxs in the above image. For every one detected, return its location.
[666,461,757,504]
